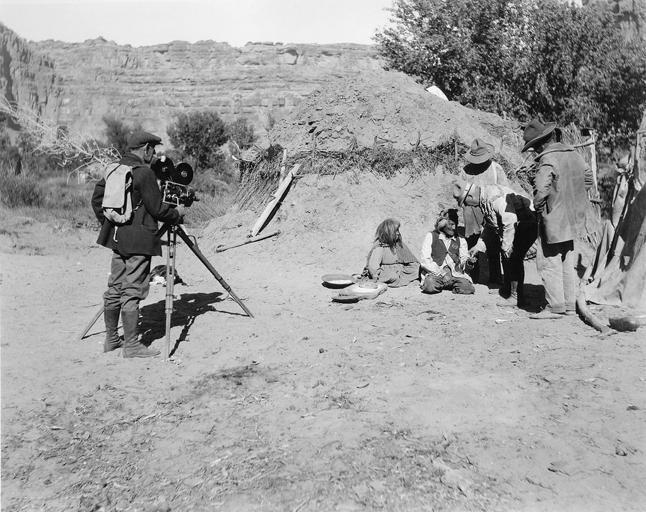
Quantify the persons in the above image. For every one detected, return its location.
[91,131,188,358]
[367,218,421,288]
[521,119,594,319]
[453,180,538,306]
[460,138,508,285]
[419,209,475,294]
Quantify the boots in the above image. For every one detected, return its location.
[496,281,523,306]
[104,308,161,358]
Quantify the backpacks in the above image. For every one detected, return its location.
[101,163,146,224]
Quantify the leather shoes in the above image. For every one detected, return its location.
[528,309,576,319]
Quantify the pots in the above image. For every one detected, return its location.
[321,273,358,285]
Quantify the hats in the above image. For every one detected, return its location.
[464,138,495,164]
[127,131,163,149]
[437,209,458,224]
[452,180,474,207]
[521,118,557,153]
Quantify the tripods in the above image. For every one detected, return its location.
[80,221,253,361]
